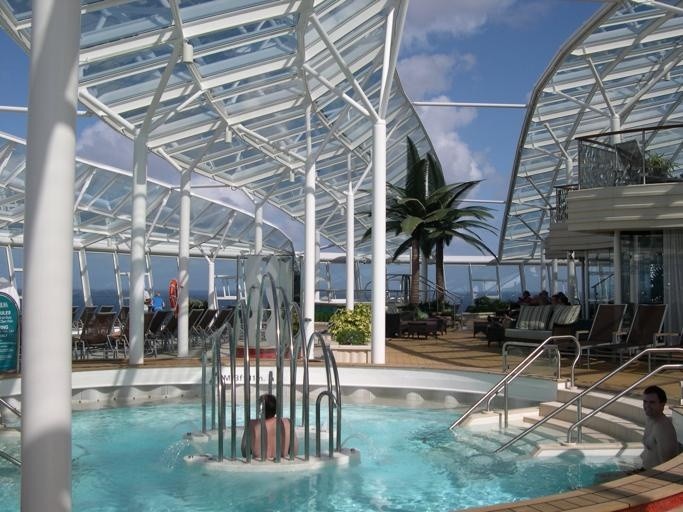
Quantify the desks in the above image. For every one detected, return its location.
[407,317,449,340]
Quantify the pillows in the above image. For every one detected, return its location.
[515,304,581,330]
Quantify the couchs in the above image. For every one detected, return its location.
[497,302,583,358]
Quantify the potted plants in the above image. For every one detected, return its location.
[326,303,372,364]
[645,152,682,178]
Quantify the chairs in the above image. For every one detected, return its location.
[611,137,682,184]
[72,305,271,359]
[554,303,682,373]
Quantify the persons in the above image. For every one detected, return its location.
[238,392,298,460]
[150,291,165,312]
[514,289,573,306]
[592,384,679,489]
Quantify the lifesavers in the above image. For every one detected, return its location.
[169,279,179,315]
